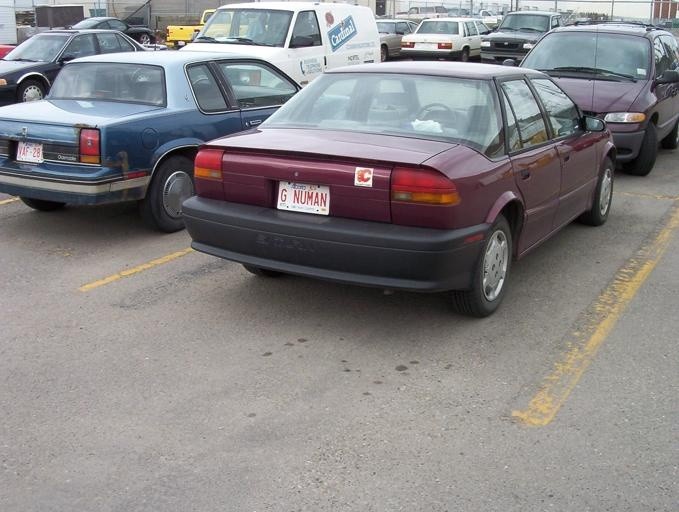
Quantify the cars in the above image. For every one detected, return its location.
[61,16,159,48]
[0,29,167,105]
[0,50,303,232]
[180,60,618,318]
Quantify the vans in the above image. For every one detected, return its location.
[180,1,382,90]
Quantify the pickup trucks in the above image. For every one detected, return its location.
[167,9,249,50]
